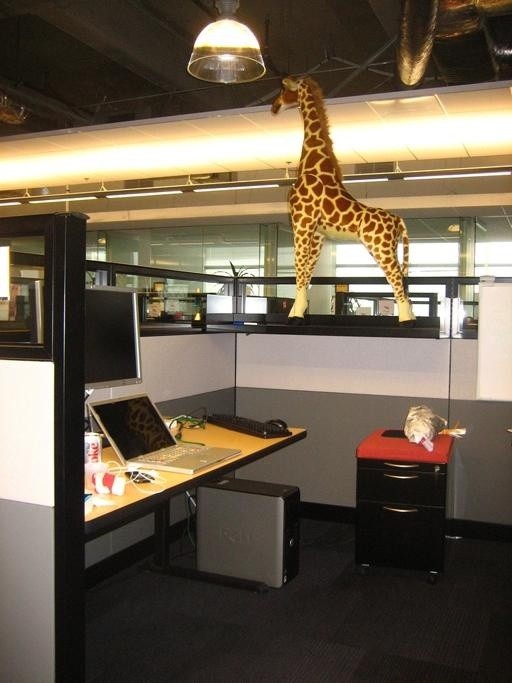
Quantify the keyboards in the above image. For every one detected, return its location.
[204,413,293,438]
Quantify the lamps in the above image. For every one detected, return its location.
[97,230,107,245]
[448,223,460,232]
[186,0,267,84]
[153,282,164,290]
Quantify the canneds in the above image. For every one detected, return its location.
[84,432,101,464]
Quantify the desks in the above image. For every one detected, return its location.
[83,415,306,593]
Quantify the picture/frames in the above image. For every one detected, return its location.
[377,299,394,317]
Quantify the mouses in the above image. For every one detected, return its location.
[267,419,287,429]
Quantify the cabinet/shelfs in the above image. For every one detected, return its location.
[355,428,454,571]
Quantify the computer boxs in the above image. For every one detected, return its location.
[195,478,302,587]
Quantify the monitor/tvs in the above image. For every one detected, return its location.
[34,280,143,390]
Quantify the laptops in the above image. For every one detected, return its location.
[87,393,241,475]
